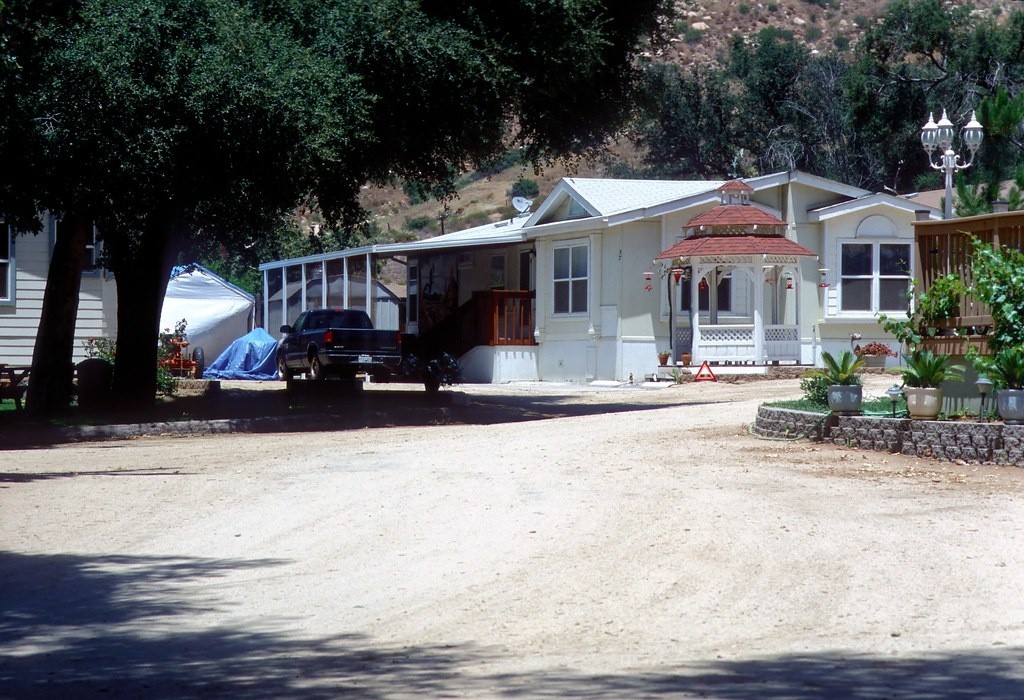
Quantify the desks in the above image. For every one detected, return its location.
[0,365,33,410]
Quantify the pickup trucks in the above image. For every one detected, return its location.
[276,309,402,383]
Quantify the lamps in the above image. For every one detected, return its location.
[975,376,993,393]
[886,385,903,402]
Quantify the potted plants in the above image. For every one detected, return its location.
[681,352,692,365]
[658,350,671,365]
[859,342,898,368]
[982,345,1024,424]
[802,350,868,412]
[886,347,966,419]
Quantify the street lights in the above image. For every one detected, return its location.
[921,107,983,219]
